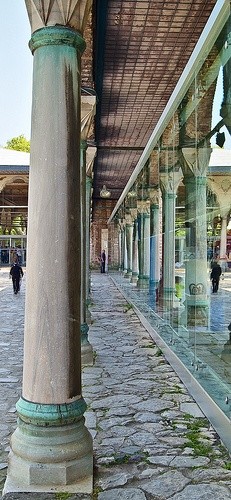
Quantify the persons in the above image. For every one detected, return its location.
[10,261,24,295]
[100,249,106,273]
[208,264,223,293]
